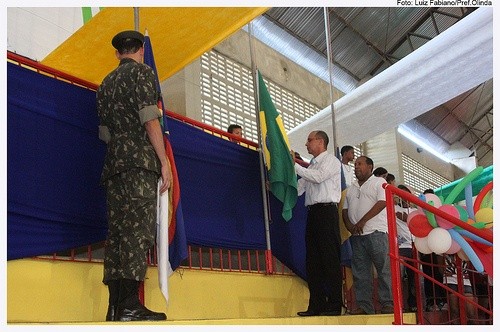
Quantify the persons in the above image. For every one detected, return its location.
[343,157,394,315]
[292,130,342,315]
[95,30,173,322]
[425,188,447,310]
[341,145,355,188]
[228,123,243,143]
[395,183,420,312]
[374,167,396,185]
[468,260,488,317]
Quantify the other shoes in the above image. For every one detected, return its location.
[425,302,440,311]
[297,306,326,315]
[350,307,366,314]
[381,304,394,314]
[437,300,449,310]
[318,305,342,315]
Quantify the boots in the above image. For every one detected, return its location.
[106,278,118,320]
[114,276,168,320]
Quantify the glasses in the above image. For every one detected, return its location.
[308,138,322,142]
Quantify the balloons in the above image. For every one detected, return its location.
[407,165,493,283]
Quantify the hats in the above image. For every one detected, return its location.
[112,30,146,48]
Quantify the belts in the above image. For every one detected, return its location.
[307,202,338,211]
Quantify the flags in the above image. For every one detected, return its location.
[257,69,297,220]
[141,32,186,302]
